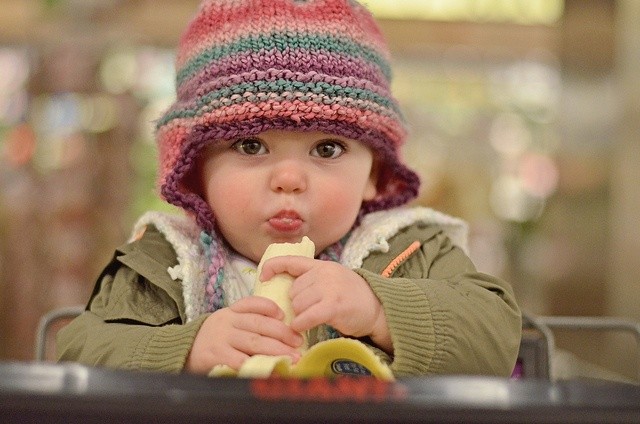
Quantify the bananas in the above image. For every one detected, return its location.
[203,236,396,381]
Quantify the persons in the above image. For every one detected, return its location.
[56,0,523,376]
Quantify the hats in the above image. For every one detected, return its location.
[154,0,421,212]
